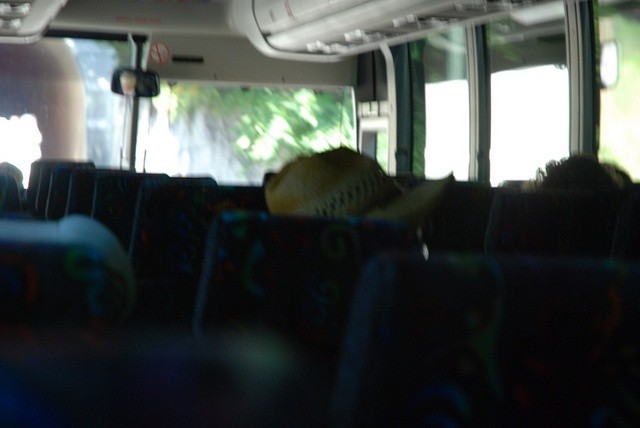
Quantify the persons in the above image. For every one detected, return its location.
[263,147,454,251]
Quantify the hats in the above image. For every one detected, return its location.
[264,146,458,232]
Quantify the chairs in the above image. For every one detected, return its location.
[26,160,40,203]
[333,253,525,426]
[484,190,632,251]
[517,259,636,427]
[194,207,354,350]
[66,167,96,216]
[307,217,415,344]
[128,176,257,320]
[45,163,73,219]
[35,160,51,213]
[90,172,169,251]
[0,168,24,210]
